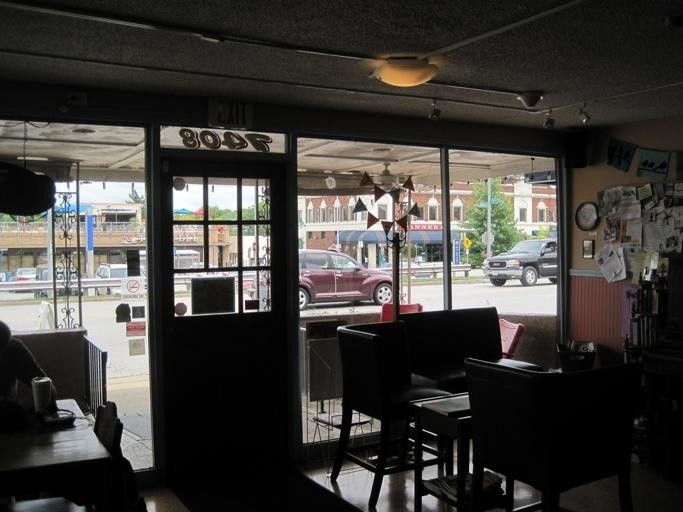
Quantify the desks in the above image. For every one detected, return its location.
[0,393,111,507]
[409,392,472,512]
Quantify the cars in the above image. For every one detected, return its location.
[298,248,394,311]
[9,267,36,293]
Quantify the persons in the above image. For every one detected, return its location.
[0,320,58,418]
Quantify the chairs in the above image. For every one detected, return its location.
[498,318,525,359]
[330,318,455,511]
[399,306,505,392]
[306,337,375,474]
[466,356,634,511]
[379,304,422,322]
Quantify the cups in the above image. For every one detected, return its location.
[31,377,52,414]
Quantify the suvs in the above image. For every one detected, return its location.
[485,238,558,287]
[97,263,147,295]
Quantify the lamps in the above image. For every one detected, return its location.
[366,56,441,89]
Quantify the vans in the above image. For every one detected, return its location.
[37,264,83,297]
